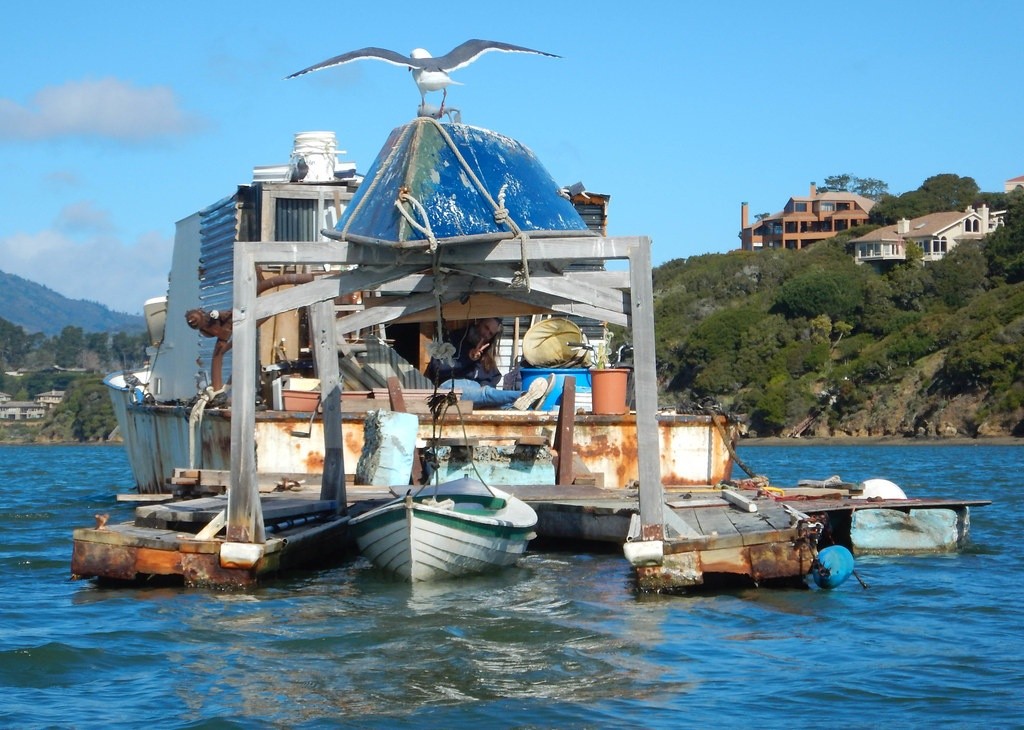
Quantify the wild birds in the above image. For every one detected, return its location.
[664,366,706,399]
[282,39,566,119]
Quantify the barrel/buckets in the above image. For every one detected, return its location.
[292,131,337,181]
[813,544,855,589]
[589,368,630,415]
[517,367,592,412]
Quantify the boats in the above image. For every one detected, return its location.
[101,131,748,543]
[319,121,599,248]
[348,473,538,582]
